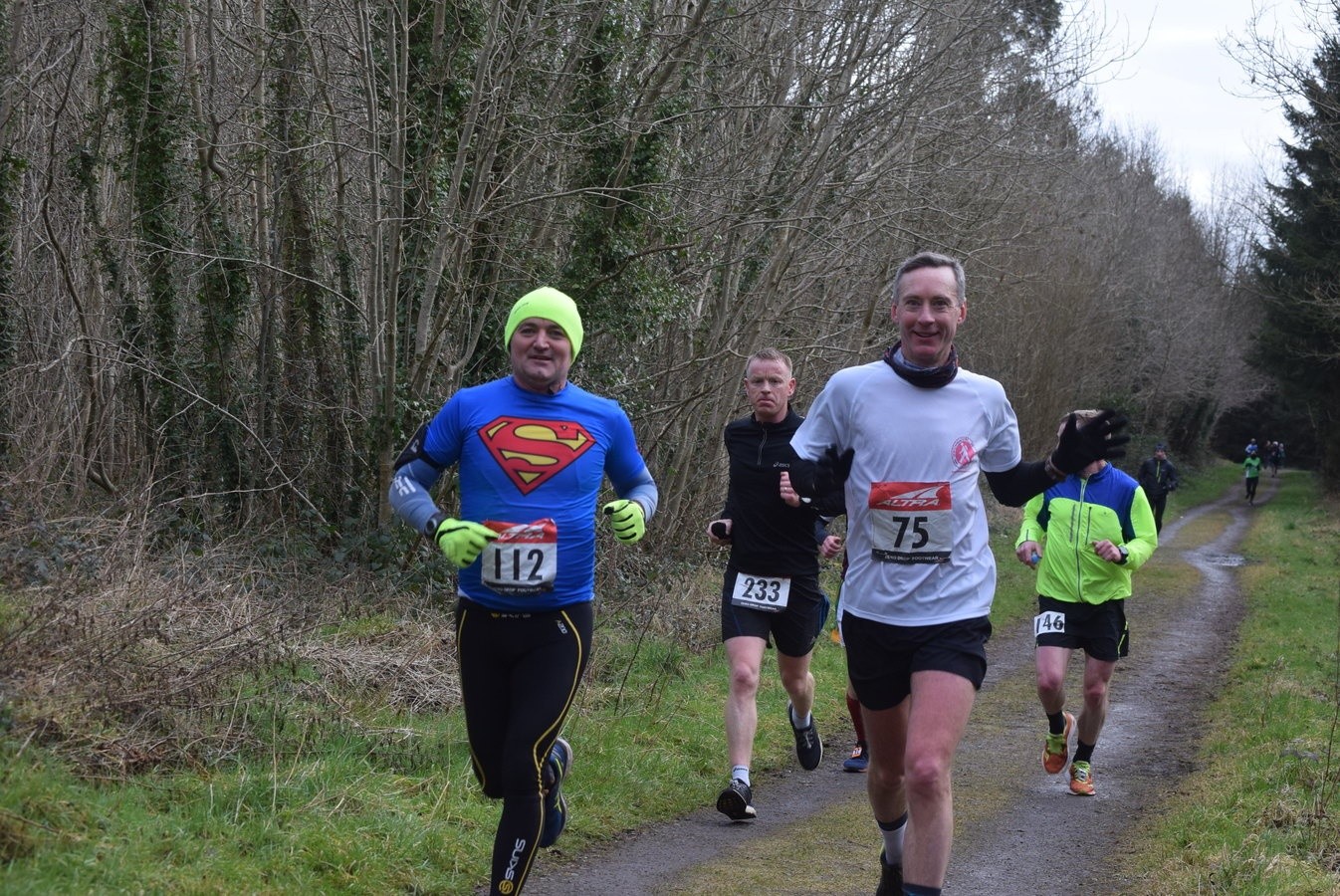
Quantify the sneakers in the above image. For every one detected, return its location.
[718,779,757,823]
[1067,759,1097,796]
[844,744,871,773]
[787,699,823,771]
[875,843,903,896]
[538,737,572,848]
[1043,708,1077,774]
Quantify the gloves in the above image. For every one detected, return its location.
[1050,408,1131,475]
[814,442,856,496]
[434,517,499,568]
[1167,484,1176,491]
[601,499,645,544]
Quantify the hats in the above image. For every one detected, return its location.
[505,285,584,367]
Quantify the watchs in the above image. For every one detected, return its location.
[797,497,812,509]
[424,513,448,542]
[1113,547,1130,565]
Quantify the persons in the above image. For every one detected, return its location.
[1137,443,1178,538]
[1242,437,1286,507]
[787,251,1131,896]
[814,506,870,772]
[707,348,839,820]
[1015,408,1158,796]
[388,288,658,896]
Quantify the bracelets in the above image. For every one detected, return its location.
[1045,456,1066,484]
